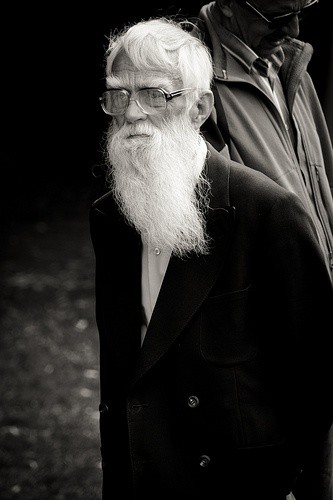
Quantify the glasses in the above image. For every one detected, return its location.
[99,86,194,117]
[242,0,319,25]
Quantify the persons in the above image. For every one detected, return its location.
[88,20,333,500]
[187,0,333,280]
